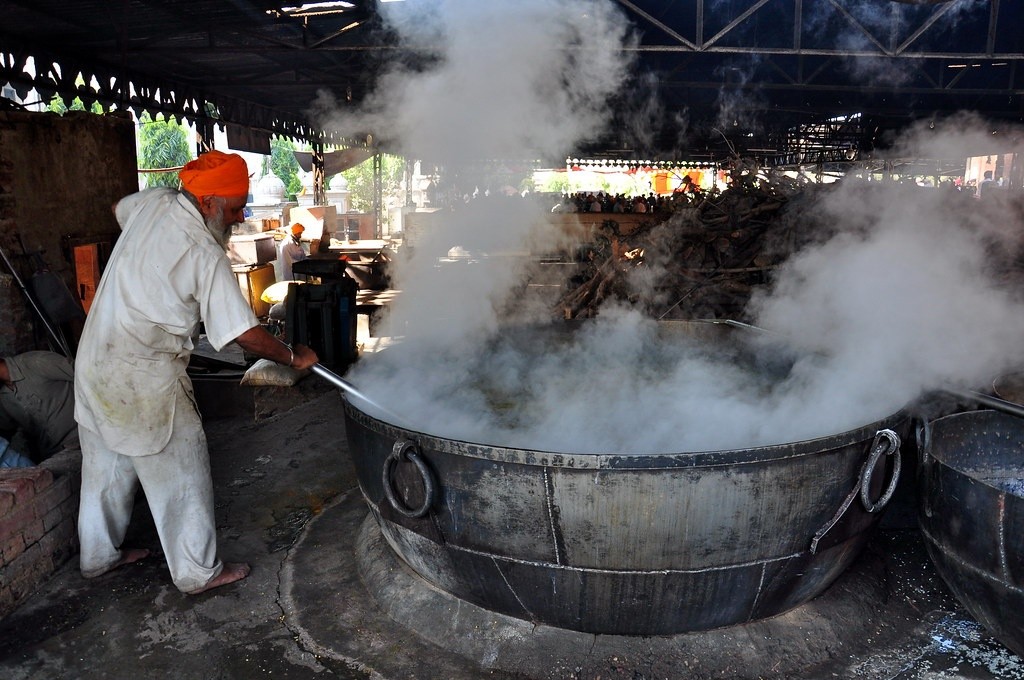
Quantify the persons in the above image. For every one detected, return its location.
[318,231,341,256]
[977,169,999,198]
[922,179,934,186]
[966,181,971,186]
[280,223,312,281]
[672,189,705,209]
[589,216,632,266]
[74,147,319,593]
[473,192,672,214]
[0,350,79,462]
[955,176,962,184]
[946,176,953,182]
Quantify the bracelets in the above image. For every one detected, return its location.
[276,346,293,367]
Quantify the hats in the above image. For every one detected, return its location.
[291,223,305,235]
[178,151,249,198]
